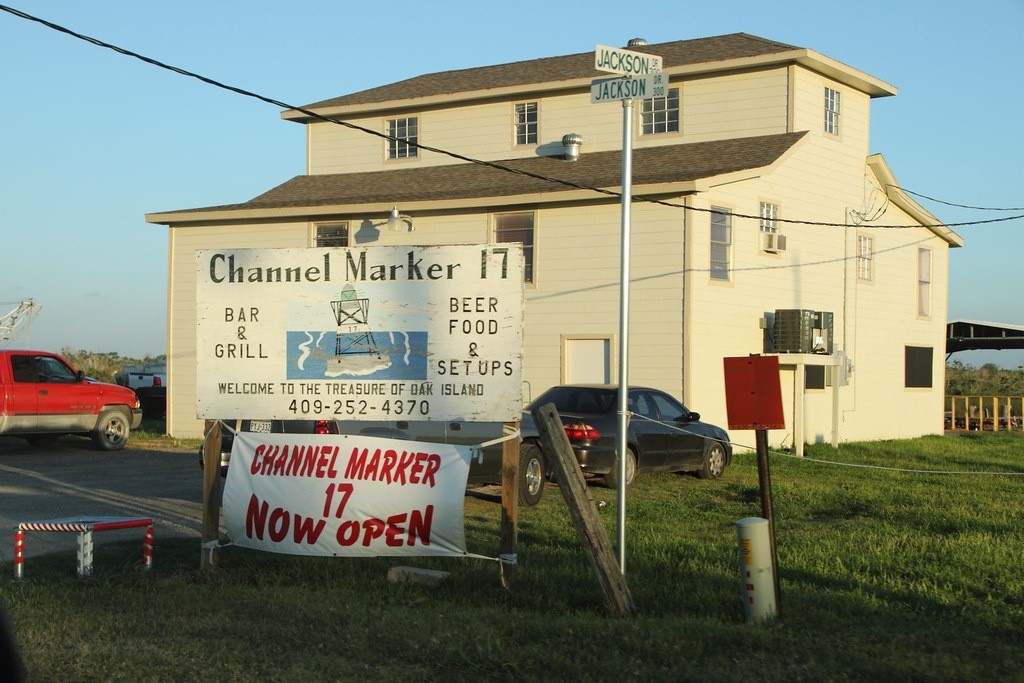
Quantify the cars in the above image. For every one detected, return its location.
[524,384,734,492]
[201,413,546,507]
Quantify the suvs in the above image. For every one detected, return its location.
[0,351,144,451]
